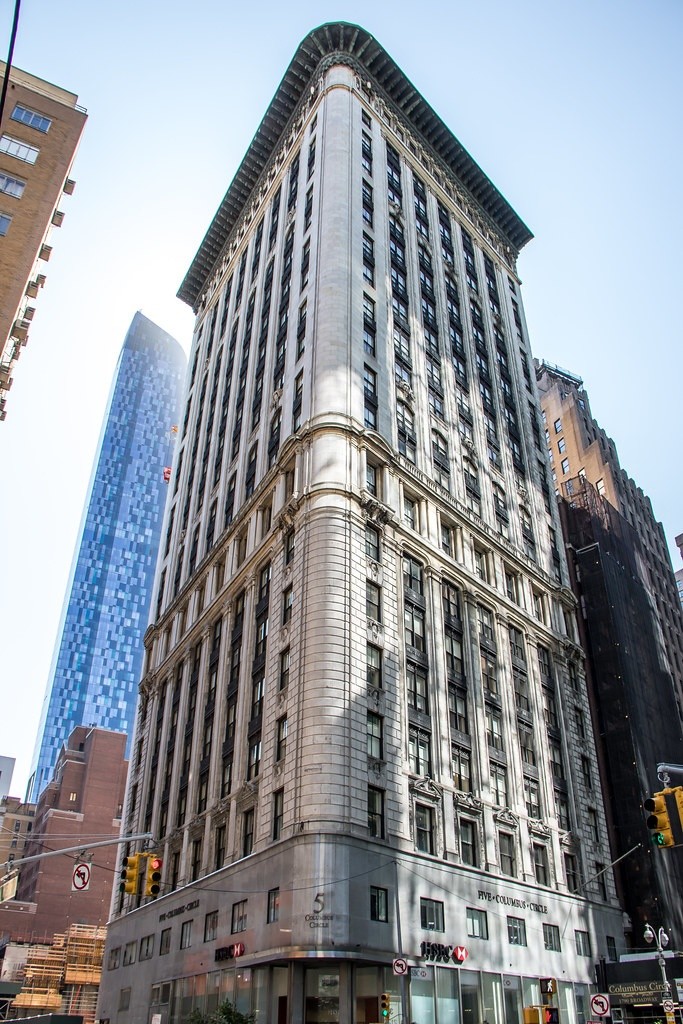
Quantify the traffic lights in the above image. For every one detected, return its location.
[540,977,557,994]
[381,991,391,1018]
[120,855,139,895]
[643,795,674,850]
[143,856,162,897]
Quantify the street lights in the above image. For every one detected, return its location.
[642,923,677,1024]
